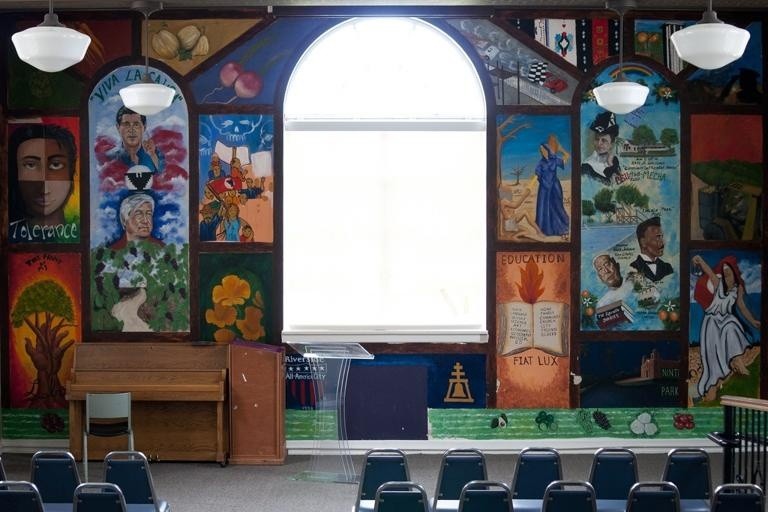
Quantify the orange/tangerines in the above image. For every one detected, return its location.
[536,411,557,431]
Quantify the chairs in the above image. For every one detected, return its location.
[71,480,128,512]
[708,481,766,512]
[349,447,414,512]
[0,456,47,512]
[623,480,683,511]
[660,445,716,511]
[540,478,598,512]
[79,392,134,485]
[587,446,641,511]
[512,445,566,512]
[430,445,488,512]
[102,449,173,511]
[457,478,515,512]
[30,447,82,505]
[371,478,430,511]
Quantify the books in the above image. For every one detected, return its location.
[498,301,571,357]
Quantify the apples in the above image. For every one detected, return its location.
[40,412,64,432]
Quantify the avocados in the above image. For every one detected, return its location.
[491,413,509,429]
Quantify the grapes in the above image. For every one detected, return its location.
[593,410,610,428]
[577,411,593,434]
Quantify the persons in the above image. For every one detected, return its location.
[593,253,641,310]
[199,153,273,243]
[96,194,182,288]
[97,105,165,184]
[693,254,760,396]
[581,111,620,185]
[630,216,673,282]
[535,143,571,235]
[11,124,80,243]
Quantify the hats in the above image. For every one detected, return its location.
[590,111,618,135]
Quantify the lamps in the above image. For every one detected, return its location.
[590,0,651,115]
[117,1,175,117]
[669,0,753,70]
[8,1,91,72]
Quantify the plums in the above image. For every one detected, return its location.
[673,413,695,430]
